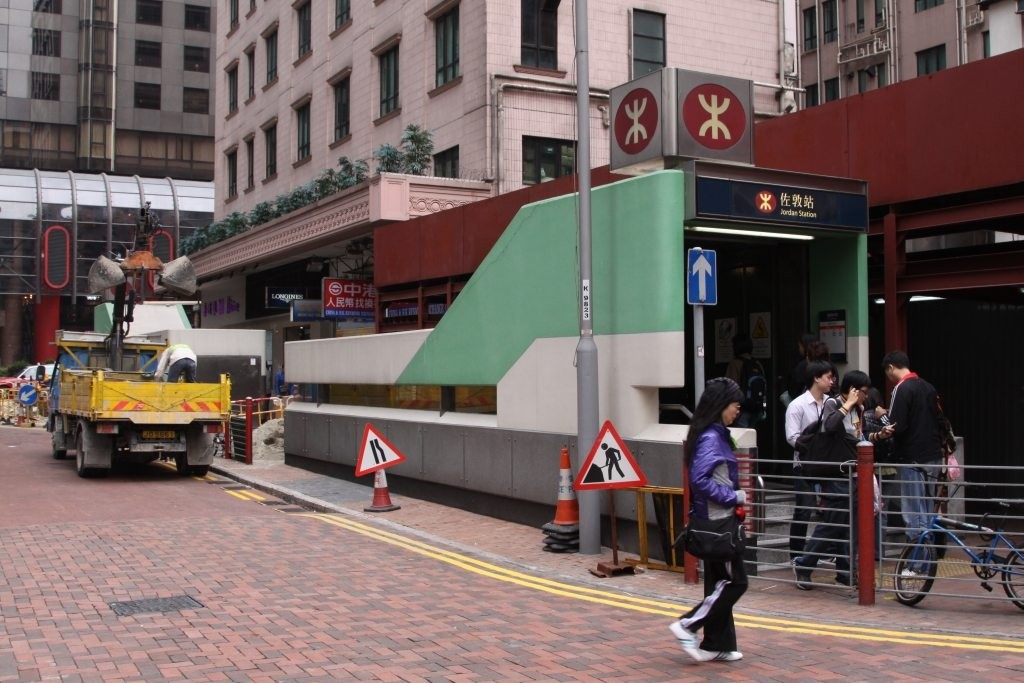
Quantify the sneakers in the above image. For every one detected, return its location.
[670,621,701,662]
[698,648,743,662]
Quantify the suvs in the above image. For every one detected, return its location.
[0,364,60,402]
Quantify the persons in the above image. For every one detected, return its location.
[155,344,197,381]
[668,334,950,665]
[274,364,300,401]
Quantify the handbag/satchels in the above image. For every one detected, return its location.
[686,508,746,562]
[945,453,961,480]
[796,420,848,468]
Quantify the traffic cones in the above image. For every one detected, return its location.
[364,471,400,513]
[540,448,580,554]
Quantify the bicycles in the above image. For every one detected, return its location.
[893,461,1024,611]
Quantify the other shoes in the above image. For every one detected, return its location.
[900,567,928,579]
[835,574,859,589]
[792,556,812,589]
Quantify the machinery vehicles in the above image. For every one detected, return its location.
[46,250,233,479]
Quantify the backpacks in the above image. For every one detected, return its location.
[737,355,768,420]
